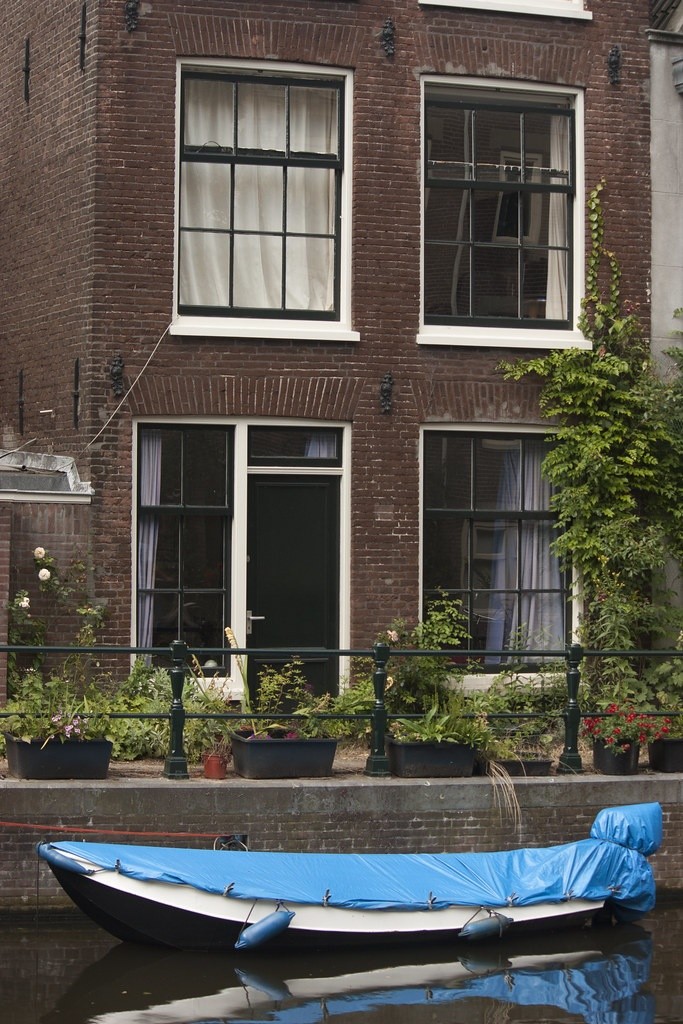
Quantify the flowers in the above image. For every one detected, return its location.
[225,628,330,740]
[40,709,90,743]
[581,702,671,755]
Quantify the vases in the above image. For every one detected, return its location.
[229,729,337,780]
[592,739,641,777]
[648,737,683,773]
[5,732,113,780]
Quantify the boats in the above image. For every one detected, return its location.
[31,800,666,950]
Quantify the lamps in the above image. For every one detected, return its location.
[607,46,624,84]
[124,0,140,30]
[379,369,395,410]
[109,350,125,395]
[381,17,396,56]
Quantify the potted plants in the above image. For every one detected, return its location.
[493,733,556,776]
[199,720,232,779]
[385,705,483,779]
